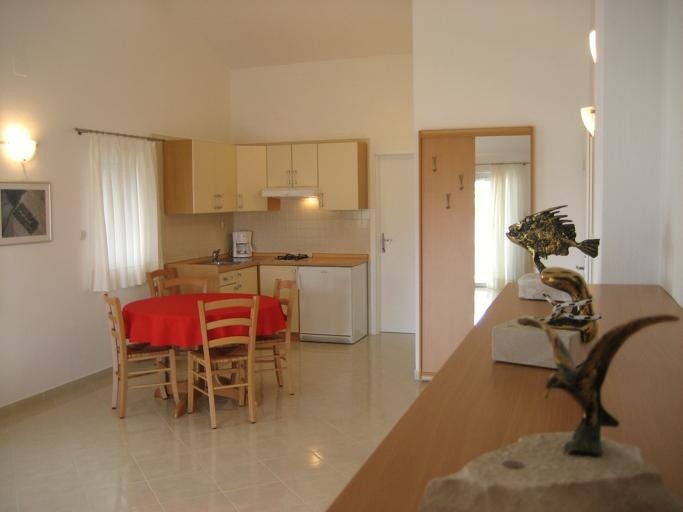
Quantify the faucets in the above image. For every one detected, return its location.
[212,248,222,262]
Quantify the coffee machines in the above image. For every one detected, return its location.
[232,230,253,258]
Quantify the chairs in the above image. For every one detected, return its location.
[255,278,296,395]
[158,277,210,394]
[102,291,180,419]
[188,296,259,429]
[145,268,177,297]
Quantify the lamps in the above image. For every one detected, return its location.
[581,105,596,135]
[589,30,596,66]
[2,140,37,162]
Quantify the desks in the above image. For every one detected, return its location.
[322,282,683,512]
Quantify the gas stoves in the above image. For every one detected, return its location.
[273,252,308,262]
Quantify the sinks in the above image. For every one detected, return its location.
[199,260,240,265]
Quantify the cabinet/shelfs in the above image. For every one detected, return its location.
[234,145,281,212]
[164,139,236,215]
[165,265,257,295]
[266,143,318,189]
[299,262,368,344]
[259,265,298,333]
[319,141,367,210]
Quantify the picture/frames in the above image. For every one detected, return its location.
[0,180,52,246]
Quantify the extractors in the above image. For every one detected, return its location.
[262,187,319,199]
